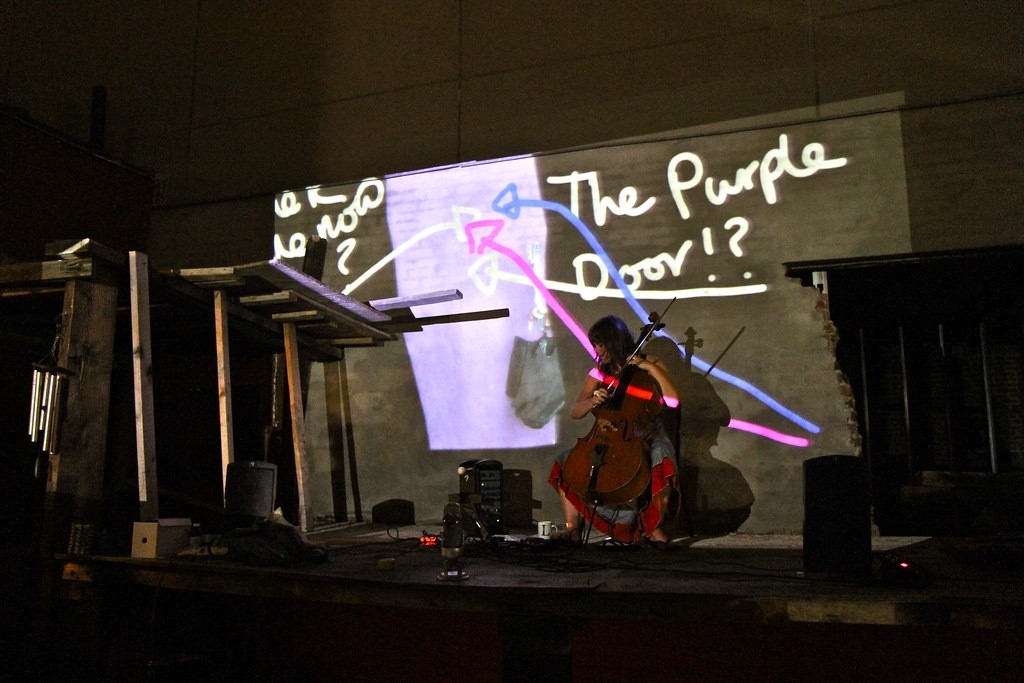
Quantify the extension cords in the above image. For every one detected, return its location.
[178,545,229,558]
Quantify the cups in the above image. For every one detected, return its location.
[538,521,558,540]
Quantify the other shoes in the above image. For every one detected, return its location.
[639,534,677,550]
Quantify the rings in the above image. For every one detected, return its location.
[596,393,599,397]
[632,356,635,360]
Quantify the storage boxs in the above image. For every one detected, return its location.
[130,518,193,559]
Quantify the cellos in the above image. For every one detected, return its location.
[559,310,667,544]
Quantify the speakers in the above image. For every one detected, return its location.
[442,502,463,548]
[799,455,873,584]
[225,460,277,533]
[460,459,504,538]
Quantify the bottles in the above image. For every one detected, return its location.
[190,523,202,548]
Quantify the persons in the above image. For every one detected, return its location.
[550,314,680,551]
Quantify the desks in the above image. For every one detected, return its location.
[0,252,344,558]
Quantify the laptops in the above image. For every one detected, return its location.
[302,237,328,279]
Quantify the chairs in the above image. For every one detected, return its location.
[579,404,693,541]
